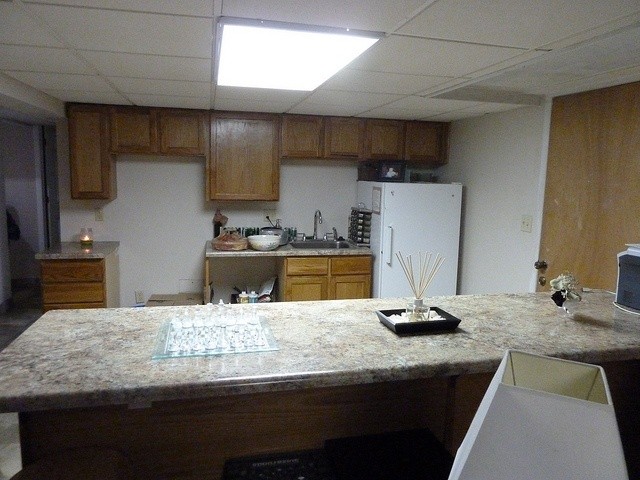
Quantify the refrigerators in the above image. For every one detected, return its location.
[354,180,464,296]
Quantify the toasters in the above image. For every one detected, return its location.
[260,227,289,247]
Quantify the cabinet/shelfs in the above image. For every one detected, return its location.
[205,108,280,203]
[328,257,372,301]
[365,118,449,169]
[107,104,208,157]
[279,113,364,159]
[33,241,121,314]
[285,256,329,301]
[64,101,118,199]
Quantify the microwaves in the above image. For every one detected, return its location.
[359,160,405,182]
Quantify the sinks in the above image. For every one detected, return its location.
[289,241,351,249]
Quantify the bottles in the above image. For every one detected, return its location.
[238,291,248,305]
[249,291,258,303]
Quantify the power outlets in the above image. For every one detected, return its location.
[95,207,104,221]
[135,289,145,303]
[520,215,533,232]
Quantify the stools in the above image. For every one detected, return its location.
[5,445,140,480]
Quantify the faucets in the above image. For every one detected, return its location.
[313,209,322,240]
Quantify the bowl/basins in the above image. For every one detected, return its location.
[247,234,278,250]
